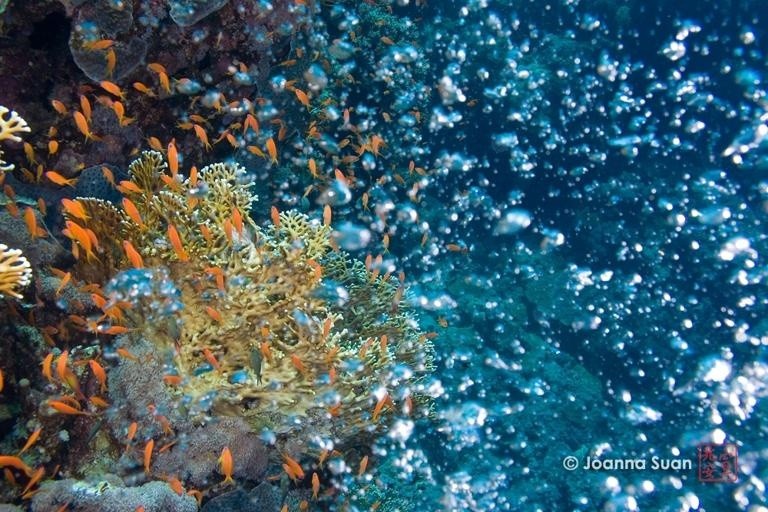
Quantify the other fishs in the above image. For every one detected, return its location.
[266,0,480,210]
